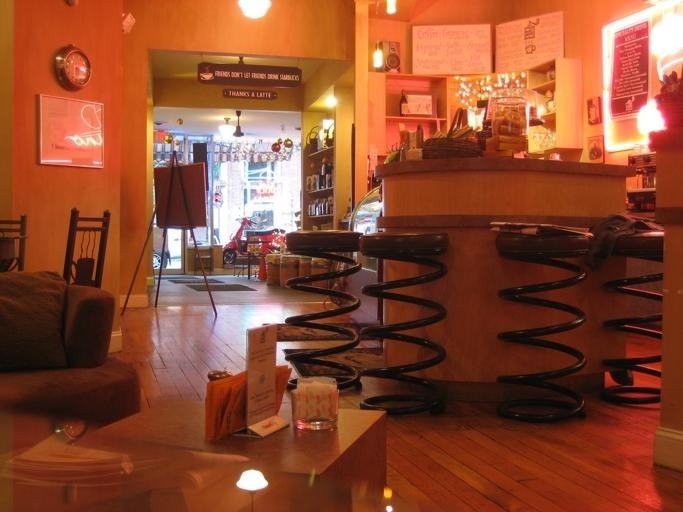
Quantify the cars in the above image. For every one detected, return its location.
[150,224,170,270]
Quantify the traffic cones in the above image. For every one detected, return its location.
[251,253,267,281]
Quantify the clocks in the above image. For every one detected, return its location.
[53,43,92,89]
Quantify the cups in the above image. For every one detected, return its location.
[291,375,341,432]
[407,148,423,160]
[490,95,529,157]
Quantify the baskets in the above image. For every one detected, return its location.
[422,107,481,159]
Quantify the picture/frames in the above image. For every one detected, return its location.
[38,95,105,169]
[404,91,437,117]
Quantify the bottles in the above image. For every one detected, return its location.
[308,197,333,216]
[400,88,410,117]
[306,162,332,191]
[400,146,406,160]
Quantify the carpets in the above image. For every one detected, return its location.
[154,274,257,292]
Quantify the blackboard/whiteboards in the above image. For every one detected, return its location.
[412,11,564,76]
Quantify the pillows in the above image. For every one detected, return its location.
[0,271,71,372]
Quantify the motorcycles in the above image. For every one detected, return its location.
[222,215,282,265]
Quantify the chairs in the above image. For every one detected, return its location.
[0,210,140,426]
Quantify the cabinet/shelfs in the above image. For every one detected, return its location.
[368,58,583,156]
[301,132,352,231]
[626,153,656,212]
